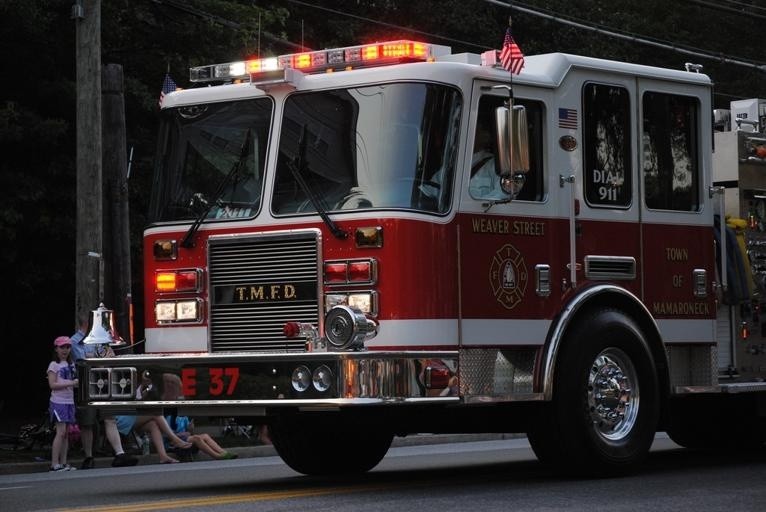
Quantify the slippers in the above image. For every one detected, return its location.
[168,442,188,449]
[159,459,179,463]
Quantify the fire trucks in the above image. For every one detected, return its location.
[76,39,766,473]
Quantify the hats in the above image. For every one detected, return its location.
[54,336,73,346]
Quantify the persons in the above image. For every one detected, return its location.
[419,124,517,211]
[70,311,138,469]
[163,415,239,460]
[115,415,193,464]
[46,336,79,473]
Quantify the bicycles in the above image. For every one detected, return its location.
[19,409,52,450]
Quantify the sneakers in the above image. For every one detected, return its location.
[49,464,76,472]
[82,457,94,468]
[222,451,238,460]
[112,454,139,467]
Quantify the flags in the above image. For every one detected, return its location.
[499,27,525,75]
